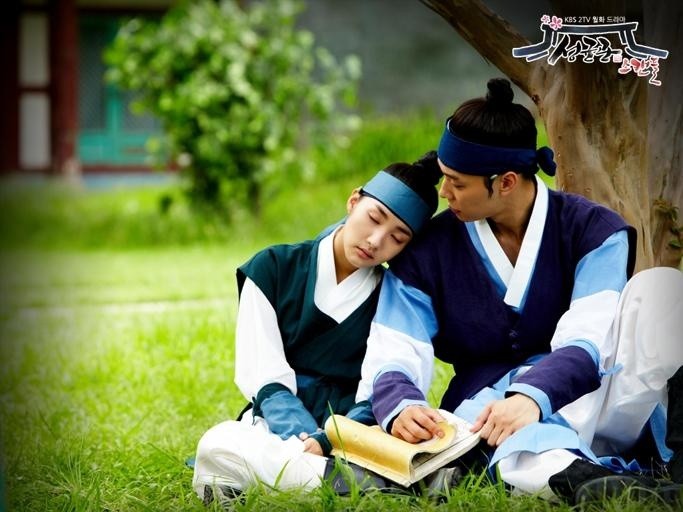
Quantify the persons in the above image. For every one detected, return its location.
[369,77,682,512]
[187,147,463,512]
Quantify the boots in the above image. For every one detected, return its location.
[665,366,683,484]
[549,459,683,512]
[323,459,462,506]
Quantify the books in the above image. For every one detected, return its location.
[323,406,486,489]
